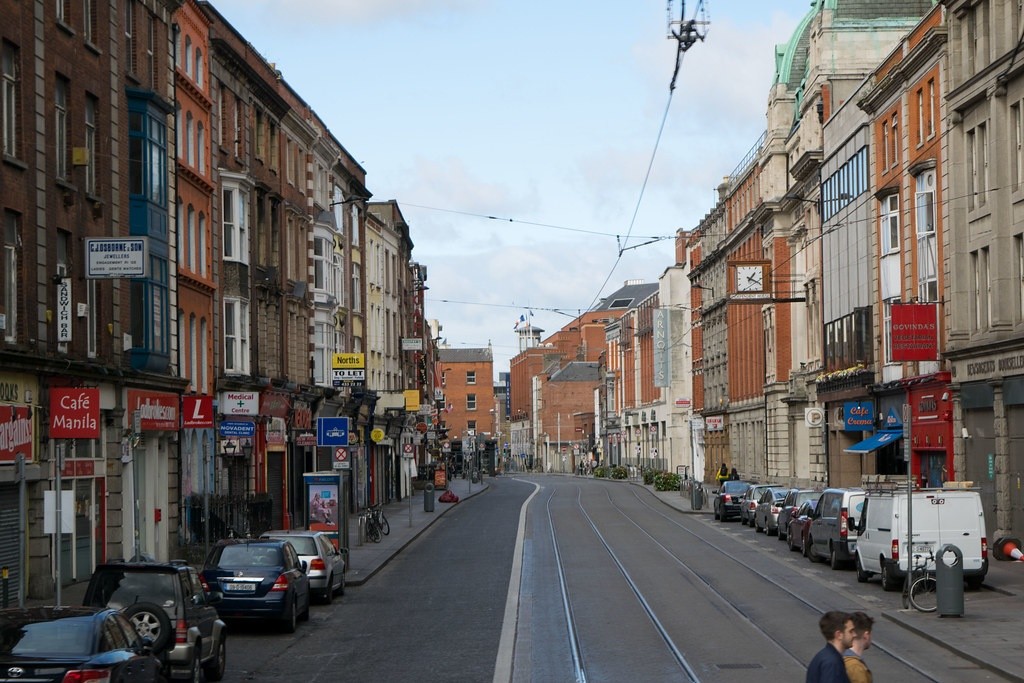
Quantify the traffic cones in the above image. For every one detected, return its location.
[996,538,1024,563]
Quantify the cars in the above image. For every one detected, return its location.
[198,538,310,632]
[740,484,784,527]
[258,529,349,604]
[786,499,819,558]
[712,480,760,522]
[754,487,799,536]
[0,607,170,682]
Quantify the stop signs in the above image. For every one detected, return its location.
[416,422,428,434]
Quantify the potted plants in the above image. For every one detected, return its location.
[818,371,874,393]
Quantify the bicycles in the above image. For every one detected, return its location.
[901,550,936,613]
[361,503,390,543]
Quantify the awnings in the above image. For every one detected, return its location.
[842,428,907,455]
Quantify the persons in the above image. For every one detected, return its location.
[715,463,729,488]
[311,493,335,526]
[580,458,598,476]
[804,610,878,683]
[728,467,740,481]
[448,464,457,481]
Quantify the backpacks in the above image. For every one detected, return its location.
[720,468,727,476]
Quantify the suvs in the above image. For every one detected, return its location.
[805,487,866,570]
[777,489,823,540]
[82,559,227,683]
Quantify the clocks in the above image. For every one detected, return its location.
[726,260,773,303]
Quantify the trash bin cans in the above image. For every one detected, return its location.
[935,544,965,616]
[129,554,155,562]
[691,481,702,509]
[424,483,435,512]
[472,467,477,483]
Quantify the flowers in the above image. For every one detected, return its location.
[815,363,870,383]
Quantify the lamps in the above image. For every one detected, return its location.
[692,283,714,298]
[241,437,253,462]
[625,325,640,331]
[822,193,852,202]
[329,194,362,206]
[217,437,237,466]
[787,194,818,204]
[120,427,140,448]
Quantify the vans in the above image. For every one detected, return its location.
[848,475,990,592]
